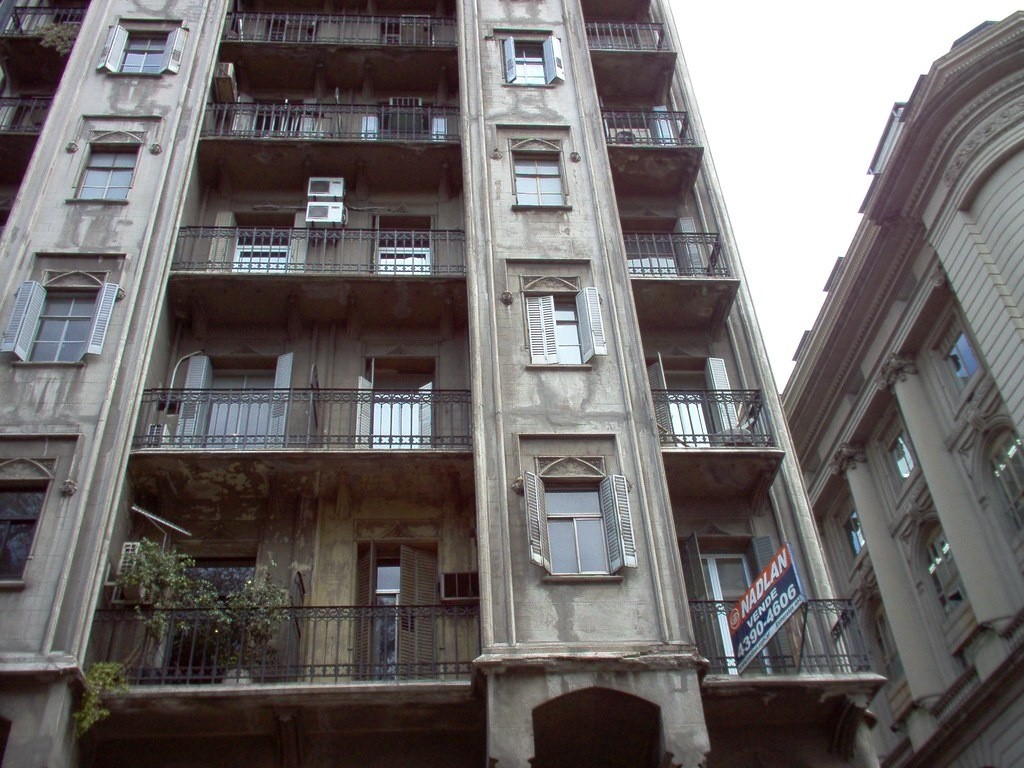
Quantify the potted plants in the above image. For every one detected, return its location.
[191,580,294,686]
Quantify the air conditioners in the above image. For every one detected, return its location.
[610,128,653,146]
[214,61,238,102]
[146,422,171,448]
[116,540,145,601]
[307,177,345,201]
[305,202,344,229]
